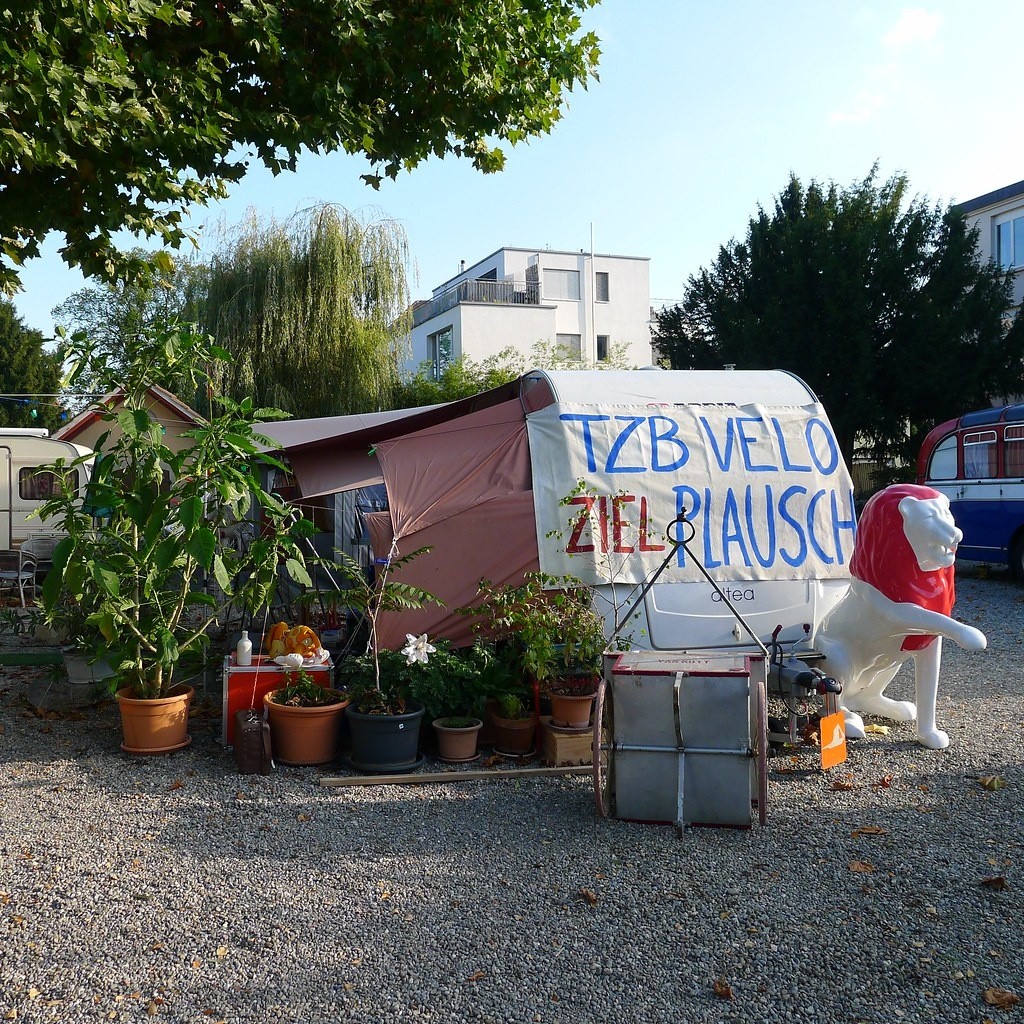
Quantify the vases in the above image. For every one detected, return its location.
[431,718,484,763]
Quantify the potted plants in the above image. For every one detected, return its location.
[1,582,120,688]
[452,566,607,735]
[22,306,324,762]
[294,543,450,775]
[472,625,548,764]
[261,666,350,767]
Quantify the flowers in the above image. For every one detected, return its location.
[402,628,482,728]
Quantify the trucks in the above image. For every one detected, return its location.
[0,427,93,596]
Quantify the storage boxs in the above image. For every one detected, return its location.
[219,651,338,752]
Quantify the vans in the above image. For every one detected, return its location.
[915,402,1023,591]
